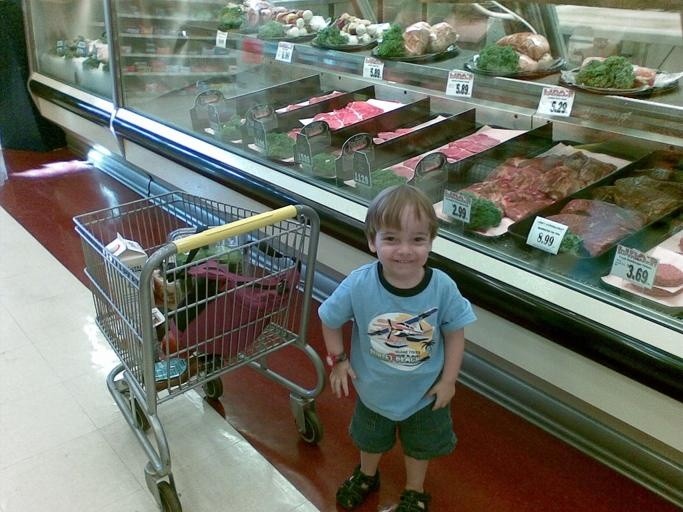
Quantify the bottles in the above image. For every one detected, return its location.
[149,354,222,392]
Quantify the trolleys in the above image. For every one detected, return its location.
[436,124,616,269]
[70,189,330,511]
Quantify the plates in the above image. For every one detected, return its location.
[311,26,384,54]
[260,20,329,39]
[466,55,565,80]
[370,41,460,62]
[559,67,667,95]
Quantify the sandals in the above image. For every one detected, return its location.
[335,462,384,512]
[394,488,432,512]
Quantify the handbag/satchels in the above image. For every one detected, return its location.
[164,229,302,360]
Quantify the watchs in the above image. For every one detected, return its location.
[325,351,348,367]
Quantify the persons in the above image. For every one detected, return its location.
[317,183,477,512]
